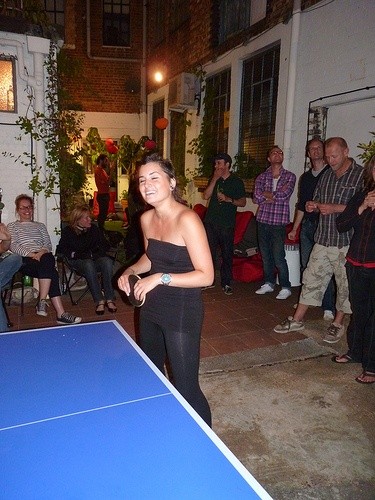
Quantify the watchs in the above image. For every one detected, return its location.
[161,272,172,287]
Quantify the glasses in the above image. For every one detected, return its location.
[18,205,32,210]
[270,150,282,154]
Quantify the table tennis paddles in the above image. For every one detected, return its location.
[128,273,146,307]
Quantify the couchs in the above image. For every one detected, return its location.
[192,204,265,284]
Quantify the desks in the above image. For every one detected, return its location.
[0,320,275,500]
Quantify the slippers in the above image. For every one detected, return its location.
[331,353,361,363]
[355,371,375,384]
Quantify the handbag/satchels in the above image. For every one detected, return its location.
[2,282,39,307]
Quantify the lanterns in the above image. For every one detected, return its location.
[144,140,156,150]
[106,139,119,154]
[155,115,169,130]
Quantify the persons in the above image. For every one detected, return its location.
[332,152,375,385]
[0,221,23,332]
[93,155,115,233]
[274,137,370,344]
[114,153,214,429]
[253,146,296,299]
[286,139,338,321]
[196,153,246,296]
[57,207,120,315]
[9,194,83,324]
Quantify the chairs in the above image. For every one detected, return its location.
[1,221,124,326]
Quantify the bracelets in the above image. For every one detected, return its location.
[231,198,234,204]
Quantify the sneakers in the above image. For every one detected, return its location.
[35,299,50,316]
[56,312,82,325]
[322,322,345,344]
[273,315,304,333]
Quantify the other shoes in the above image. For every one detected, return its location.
[255,283,274,294]
[323,310,334,320]
[276,289,291,299]
[223,285,232,295]
[95,299,105,315]
[105,300,117,313]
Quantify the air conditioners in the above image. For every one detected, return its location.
[168,72,195,110]
